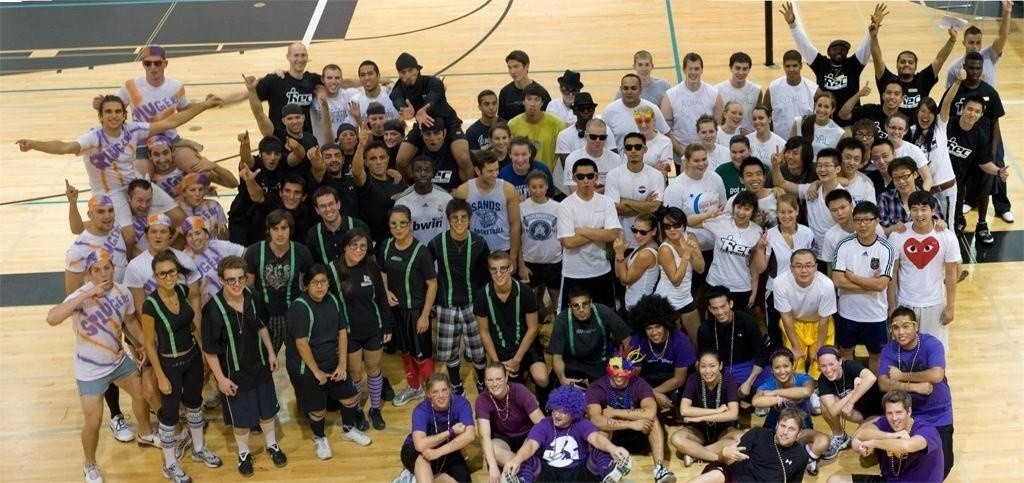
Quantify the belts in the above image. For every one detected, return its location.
[929,178,955,194]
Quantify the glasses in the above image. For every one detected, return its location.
[388,220,410,229]
[348,243,368,251]
[624,144,645,152]
[919,109,932,114]
[662,222,682,230]
[888,125,906,133]
[143,58,165,67]
[816,163,838,169]
[449,215,469,223]
[310,278,331,287]
[791,262,816,272]
[567,298,590,309]
[855,132,875,140]
[574,172,597,180]
[892,170,915,182]
[154,268,178,279]
[585,131,608,140]
[561,88,579,95]
[222,274,248,286]
[853,215,877,224]
[574,106,596,111]
[634,118,652,124]
[630,225,654,236]
[487,263,511,273]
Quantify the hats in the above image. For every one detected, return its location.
[395,53,423,72]
[827,40,850,56]
[570,93,598,110]
[558,69,584,89]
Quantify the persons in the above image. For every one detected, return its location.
[10,1,1016,483]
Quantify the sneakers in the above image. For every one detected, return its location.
[178,413,208,428]
[354,409,369,431]
[251,423,261,433]
[136,427,162,450]
[975,219,994,248]
[1001,211,1014,222]
[808,393,822,415]
[312,434,332,460]
[476,380,486,394]
[391,469,414,483]
[820,431,852,460]
[684,455,703,467]
[339,424,372,446]
[192,446,222,468]
[806,460,817,474]
[451,380,466,399]
[501,469,519,483]
[238,450,255,478]
[162,463,191,482]
[265,443,287,468]
[109,414,135,442]
[392,384,425,407]
[653,459,678,482]
[670,407,683,422]
[368,407,385,430]
[202,388,222,409]
[381,377,394,401]
[83,461,103,483]
[963,202,976,214]
[600,454,634,483]
[755,407,766,416]
[954,216,966,237]
[538,308,548,323]
[173,428,192,462]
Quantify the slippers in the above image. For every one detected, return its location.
[944,270,969,284]
